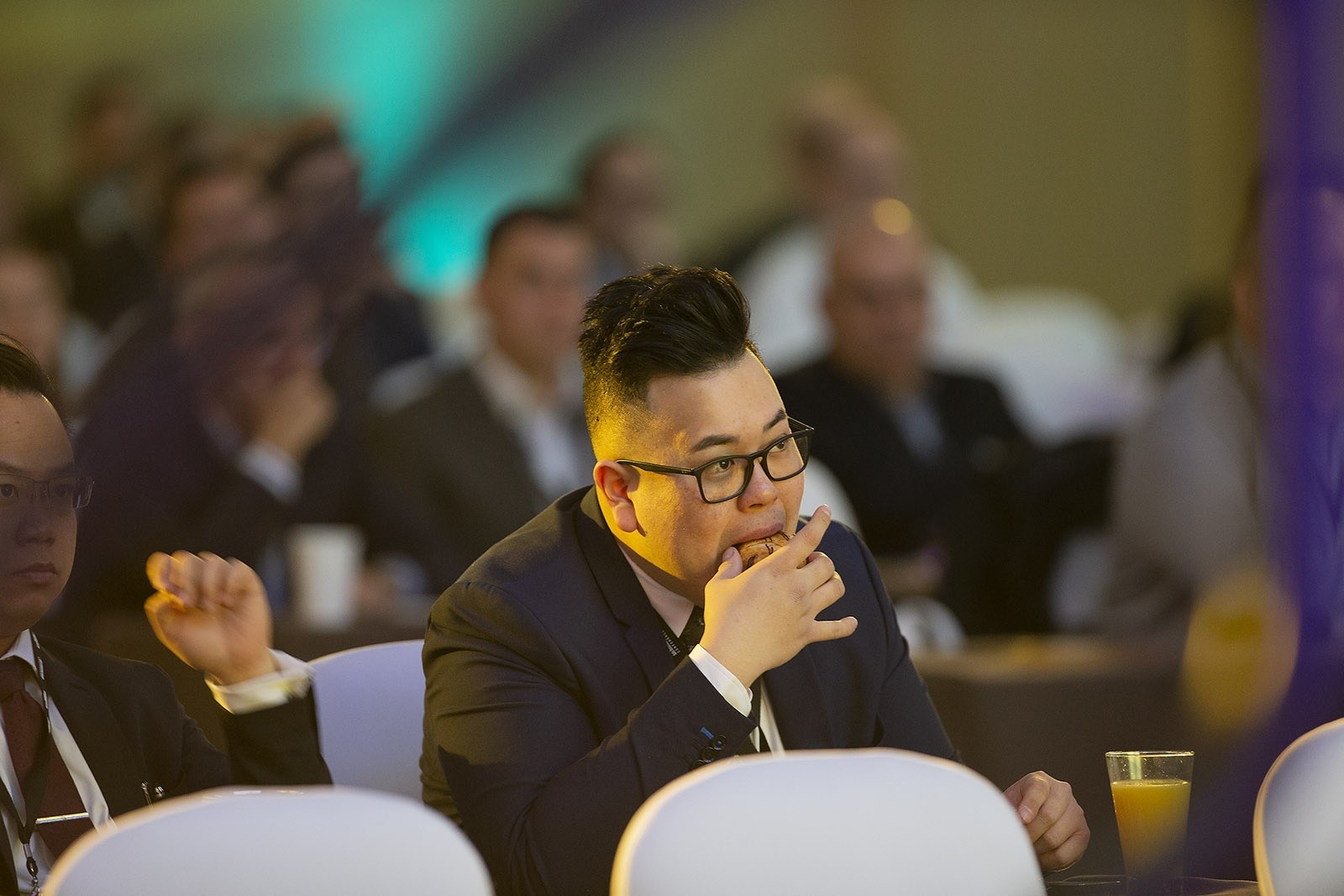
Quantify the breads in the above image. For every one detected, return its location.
[736,530,809,570]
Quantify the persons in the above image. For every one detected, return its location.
[0,330,334,896]
[421,262,1090,896]
[0,52,1273,647]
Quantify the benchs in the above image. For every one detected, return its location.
[610,748,1047,896]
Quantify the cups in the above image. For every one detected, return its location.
[1105,748,1194,892]
[285,523,361,633]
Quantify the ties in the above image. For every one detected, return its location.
[0,655,96,859]
[678,606,771,755]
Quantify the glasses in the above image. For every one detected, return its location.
[0,473,94,511]
[614,418,816,504]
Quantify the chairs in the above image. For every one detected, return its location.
[305,639,426,802]
[39,788,496,896]
[1253,719,1344,896]
